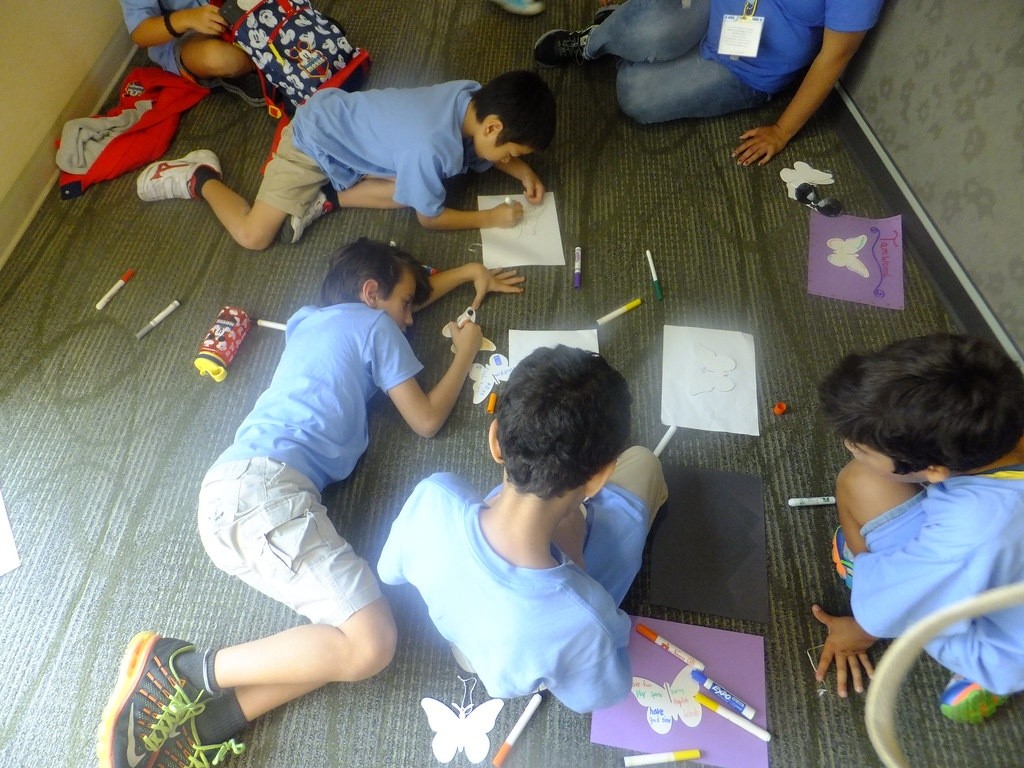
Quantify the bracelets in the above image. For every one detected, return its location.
[164,10,185,38]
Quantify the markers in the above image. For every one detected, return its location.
[492,692,543,768]
[422,264,438,276]
[250,318,286,331]
[635,623,705,672]
[690,669,756,720]
[574,247,582,290]
[504,197,514,207]
[457,309,474,328]
[95,268,136,310]
[693,693,771,742]
[134,299,182,340]
[646,249,664,301]
[623,749,701,767]
[653,425,677,459]
[596,299,642,326]
[788,496,837,507]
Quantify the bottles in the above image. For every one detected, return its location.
[195,305,250,382]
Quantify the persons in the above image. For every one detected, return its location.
[811,334,1023,725]
[376,345,668,713]
[136,69,557,250]
[534,0,884,167]
[95,238,525,768]
[120,0,278,108]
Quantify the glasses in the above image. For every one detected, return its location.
[795,183,844,218]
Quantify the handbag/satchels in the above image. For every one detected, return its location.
[219,0,372,118]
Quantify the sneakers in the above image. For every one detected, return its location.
[594,4,620,24]
[146,717,246,768]
[534,25,616,68]
[96,629,205,768]
[938,674,1010,724]
[137,149,222,202]
[832,526,854,590]
[275,187,334,244]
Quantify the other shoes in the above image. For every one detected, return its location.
[223,73,265,107]
[494,0,544,16]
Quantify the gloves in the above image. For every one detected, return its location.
[780,161,835,199]
